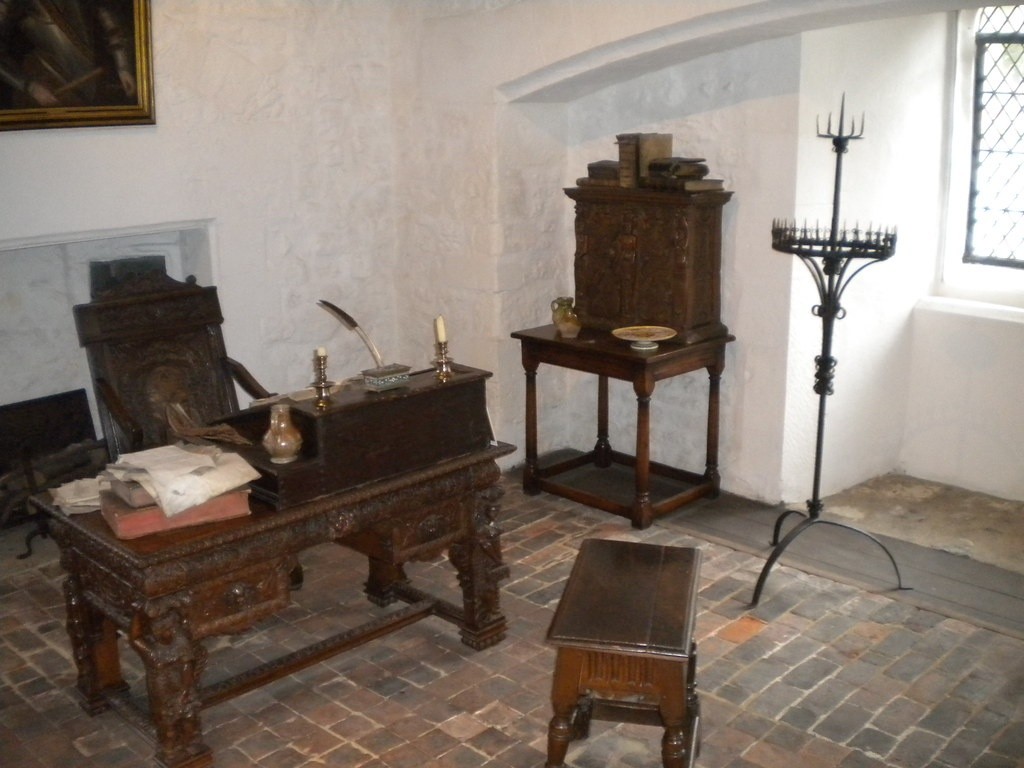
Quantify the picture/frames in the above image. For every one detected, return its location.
[0,1,156,134]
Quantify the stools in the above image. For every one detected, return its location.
[543,538,708,768]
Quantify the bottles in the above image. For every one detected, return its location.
[263,404,303,464]
[557,306,581,339]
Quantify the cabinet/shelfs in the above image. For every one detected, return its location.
[562,186,732,342]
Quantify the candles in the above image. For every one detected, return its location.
[435,313,449,345]
[315,345,327,356]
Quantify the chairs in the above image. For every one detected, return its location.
[73,255,303,594]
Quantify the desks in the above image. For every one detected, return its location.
[511,321,736,529]
[27,439,516,768]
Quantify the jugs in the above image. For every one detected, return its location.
[551,296,573,326]
